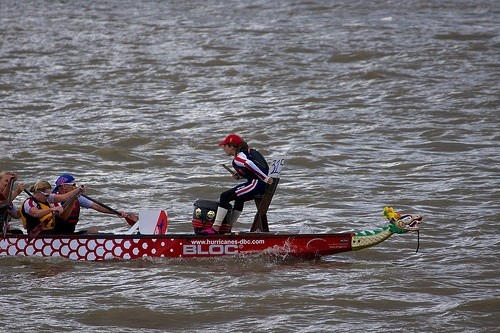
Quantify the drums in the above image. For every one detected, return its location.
[192,199,233,235]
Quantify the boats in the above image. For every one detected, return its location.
[0,207,424,263]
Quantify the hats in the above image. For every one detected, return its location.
[219,135,242,146]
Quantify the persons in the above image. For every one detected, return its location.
[202,133,274,235]
[0,171,27,236]
[52,174,129,236]
[20,180,87,236]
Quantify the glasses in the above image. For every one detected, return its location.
[40,191,50,197]
[61,183,76,187]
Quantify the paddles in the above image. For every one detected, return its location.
[81,192,139,226]
[29,185,84,239]
[24,188,32,197]
[2,173,15,241]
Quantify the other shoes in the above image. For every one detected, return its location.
[197,227,219,235]
